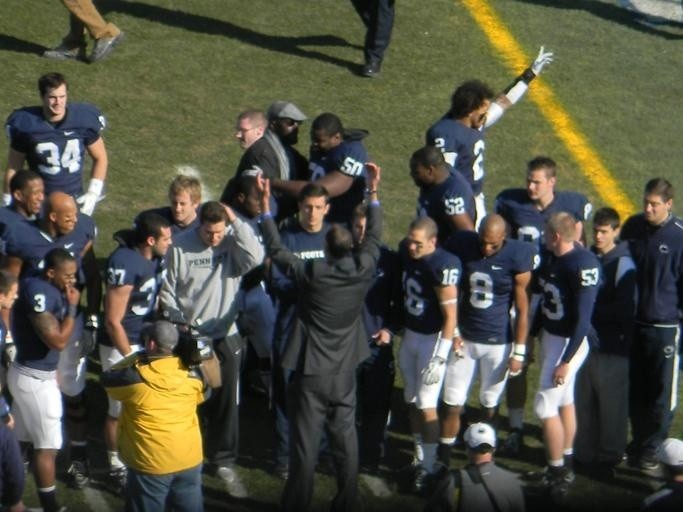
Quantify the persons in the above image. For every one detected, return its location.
[44,0,124,64]
[231,99,314,218]
[426,45,554,232]
[346,0,397,76]
[244,113,373,223]
[0,146,682,510]
[4,73,108,219]
[234,107,268,147]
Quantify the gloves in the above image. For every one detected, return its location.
[76,178,105,217]
[2,194,12,206]
[530,46,553,76]
[421,356,444,384]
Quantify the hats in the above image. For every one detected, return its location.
[269,101,306,121]
[463,422,497,448]
[140,320,178,351]
[655,438,683,466]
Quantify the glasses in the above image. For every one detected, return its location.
[286,119,303,126]
[234,125,258,131]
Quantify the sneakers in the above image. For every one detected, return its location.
[271,453,289,480]
[639,451,660,469]
[106,468,127,495]
[216,467,247,498]
[502,434,535,460]
[535,466,575,489]
[70,455,89,490]
[88,22,124,62]
[394,454,449,494]
[43,41,85,59]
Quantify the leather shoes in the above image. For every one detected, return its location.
[362,61,380,76]
